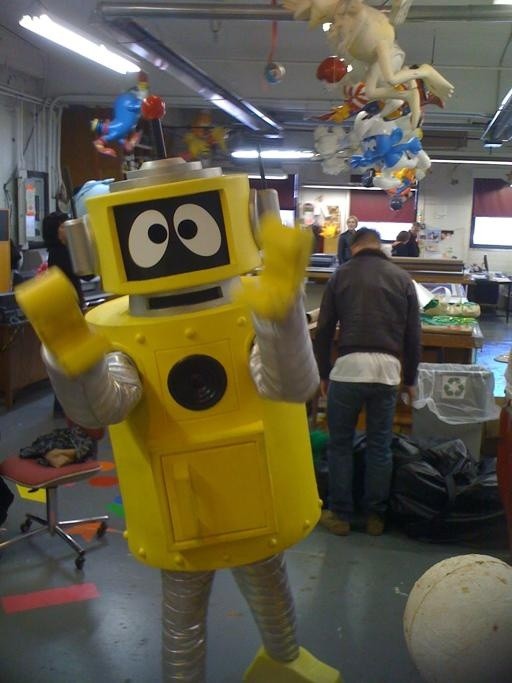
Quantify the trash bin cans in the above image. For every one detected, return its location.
[411,362,494,464]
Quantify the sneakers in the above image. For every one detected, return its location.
[366,514,385,536]
[320,508,353,536]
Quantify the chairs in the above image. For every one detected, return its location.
[1,414,110,568]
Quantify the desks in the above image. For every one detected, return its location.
[306,257,483,435]
[470,270,511,322]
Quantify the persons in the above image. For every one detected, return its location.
[18,155,343,683]
[315,215,422,536]
